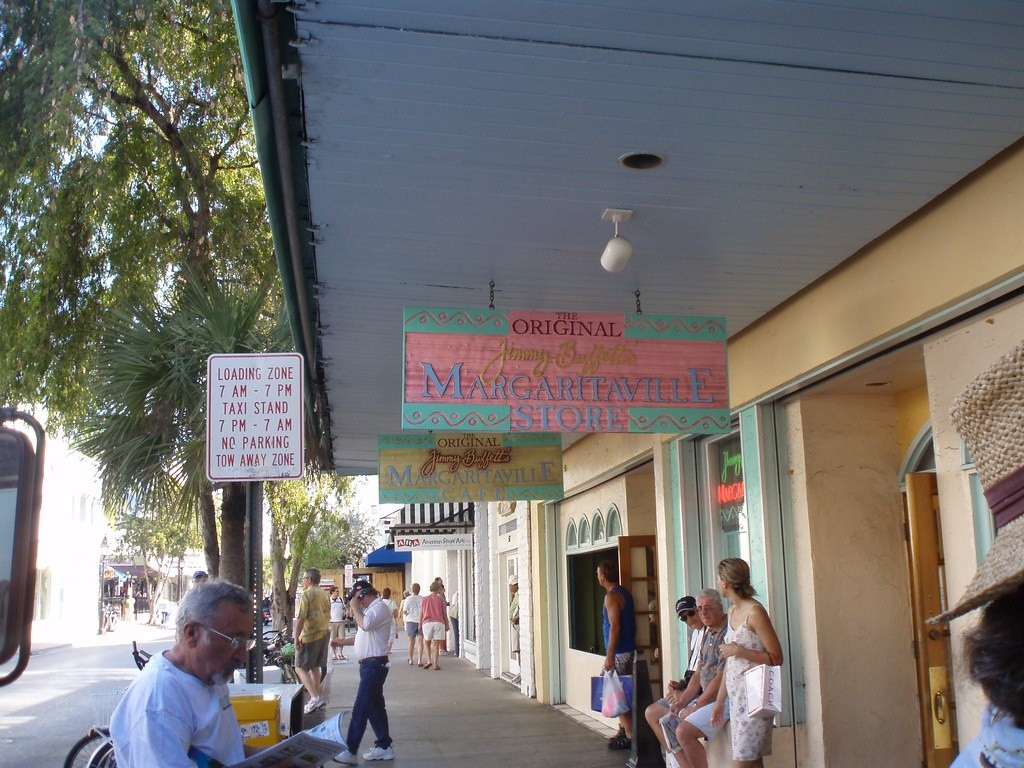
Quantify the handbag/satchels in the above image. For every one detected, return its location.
[743,651,784,718]
[589,666,633,718]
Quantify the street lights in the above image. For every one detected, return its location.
[99,537,109,635]
[180,557,186,599]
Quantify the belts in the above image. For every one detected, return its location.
[358,657,387,664]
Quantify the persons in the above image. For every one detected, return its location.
[449,591,459,657]
[417,582,450,670]
[109,580,255,768]
[645,596,710,768]
[330,586,348,660]
[659,588,730,768]
[294,568,331,713]
[125,595,135,621]
[597,560,636,750]
[399,577,450,667]
[508,575,521,683]
[924,340,1024,768]
[710,557,783,768]
[381,587,398,667]
[330,581,394,765]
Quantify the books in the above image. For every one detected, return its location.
[228,710,350,768]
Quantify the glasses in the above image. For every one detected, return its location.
[334,590,340,593]
[680,609,696,622]
[198,624,256,651]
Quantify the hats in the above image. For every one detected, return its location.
[925,339,1024,627]
[346,581,373,599]
[676,597,698,618]
[504,575,518,586]
[192,571,207,578]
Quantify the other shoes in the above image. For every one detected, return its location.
[423,662,432,669]
[434,666,441,669]
[511,673,521,685]
[408,658,413,664]
[332,655,338,660]
[417,663,423,667]
[439,650,450,655]
[338,655,345,660]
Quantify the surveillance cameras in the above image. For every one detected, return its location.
[601,237,633,273]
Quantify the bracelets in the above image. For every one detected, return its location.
[693,701,699,709]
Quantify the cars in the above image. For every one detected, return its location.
[133,596,150,611]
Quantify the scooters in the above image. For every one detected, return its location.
[262,607,270,625]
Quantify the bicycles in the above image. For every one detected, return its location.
[64,641,152,768]
[262,628,327,691]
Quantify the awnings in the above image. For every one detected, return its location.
[385,503,474,550]
[365,545,412,567]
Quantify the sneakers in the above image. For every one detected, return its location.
[610,727,626,742]
[332,750,357,764]
[608,737,631,750]
[363,745,393,760]
[304,696,324,713]
